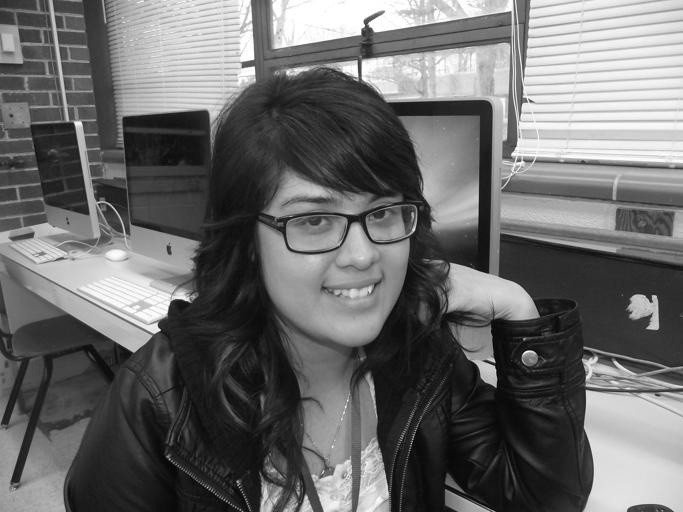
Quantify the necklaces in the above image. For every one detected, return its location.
[301,386,354,479]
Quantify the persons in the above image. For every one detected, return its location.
[57,64,596,511]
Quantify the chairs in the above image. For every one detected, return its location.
[0,276,119,492]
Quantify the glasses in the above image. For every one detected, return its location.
[252,196,428,256]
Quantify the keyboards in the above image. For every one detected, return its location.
[78,275,171,324]
[10,238,67,264]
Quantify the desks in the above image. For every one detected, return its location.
[0,222,681,511]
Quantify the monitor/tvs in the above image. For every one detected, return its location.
[386,97,502,277]
[122,110,212,300]
[30,120,114,245]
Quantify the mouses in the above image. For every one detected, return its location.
[106,249,129,261]
